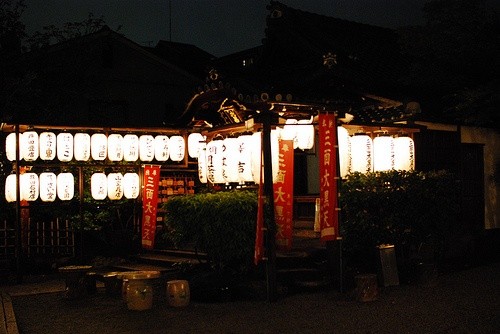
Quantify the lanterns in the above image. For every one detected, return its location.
[155,135,170,161]
[123,172,139,199]
[38,131,57,161]
[107,172,124,200]
[124,133,140,162]
[39,172,57,202]
[74,133,91,161]
[337,126,351,177]
[187,132,203,158]
[6,133,24,161]
[22,131,39,161]
[4,174,22,203]
[91,172,108,200]
[56,173,74,201]
[394,137,416,173]
[22,173,40,201]
[90,133,108,161]
[169,135,186,161]
[57,133,74,161]
[138,135,155,162]
[351,135,373,175]
[108,134,124,162]
[199,119,315,183]
[371,136,395,173]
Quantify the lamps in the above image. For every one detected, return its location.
[4,127,418,203]
